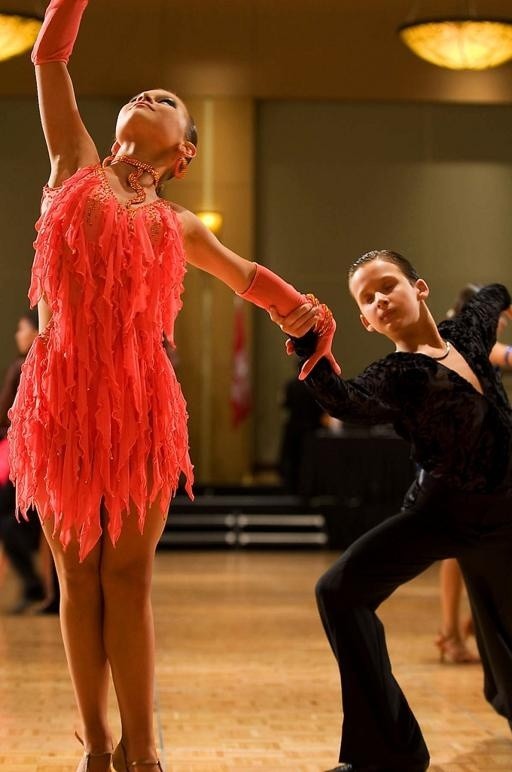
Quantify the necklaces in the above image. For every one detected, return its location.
[113,155,160,215]
[431,343,452,360]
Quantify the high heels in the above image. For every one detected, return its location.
[432,628,480,664]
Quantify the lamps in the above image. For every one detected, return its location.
[0,13,44,65]
[398,17,512,73]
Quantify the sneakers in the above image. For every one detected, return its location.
[10,590,60,616]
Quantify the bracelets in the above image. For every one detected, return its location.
[503,344,512,364]
[306,295,334,336]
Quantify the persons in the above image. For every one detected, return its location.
[437,281,511,670]
[0,307,70,617]
[0,0,347,771]
[270,242,508,772]
